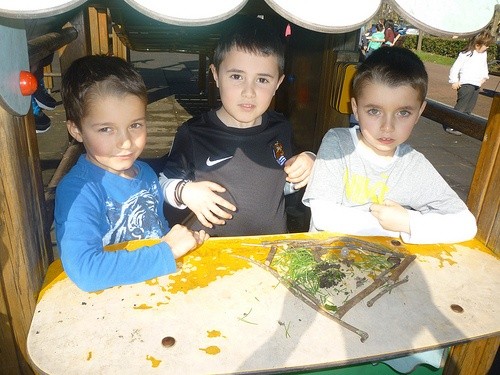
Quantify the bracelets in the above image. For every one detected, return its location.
[174,180,191,205]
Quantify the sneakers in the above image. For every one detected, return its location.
[32,69,57,110]
[31,97,52,133]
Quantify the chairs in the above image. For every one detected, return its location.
[390,34,401,46]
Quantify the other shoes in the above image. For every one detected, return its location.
[446,127,462,136]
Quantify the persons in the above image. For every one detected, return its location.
[301,46,477,244]
[55,55,210,292]
[446,30,491,135]
[32,67,57,133]
[359,18,395,57]
[159,21,316,237]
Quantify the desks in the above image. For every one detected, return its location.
[27,231,500,375]
